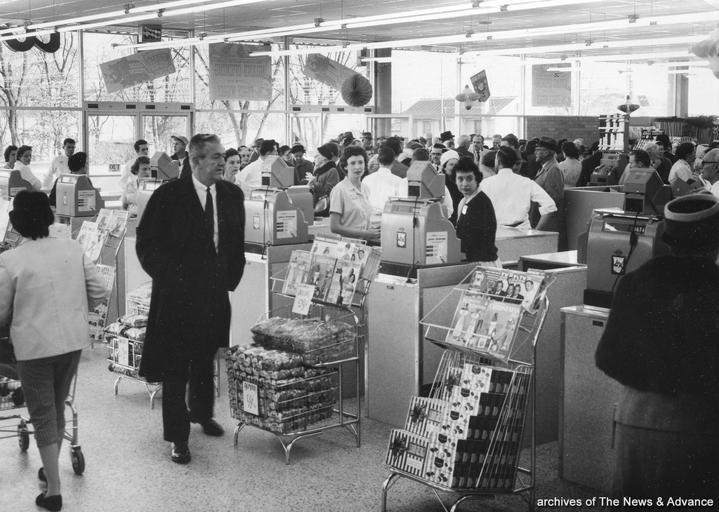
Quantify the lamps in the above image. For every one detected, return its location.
[0,0,719,127]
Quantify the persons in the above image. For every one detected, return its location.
[621,135,719,207]
[3,136,90,206]
[135,135,243,463]
[0,189,111,512]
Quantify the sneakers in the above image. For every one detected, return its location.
[36,490,63,512]
[38,466,48,482]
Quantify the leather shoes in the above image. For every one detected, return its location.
[170,439,192,465]
[189,410,225,437]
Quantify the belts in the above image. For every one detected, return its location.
[504,222,524,228]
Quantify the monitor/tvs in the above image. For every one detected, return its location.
[150,151,179,179]
[624,168,672,215]
[406,161,445,198]
[263,155,295,188]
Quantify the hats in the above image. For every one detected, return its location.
[170,134,189,146]
[316,142,339,161]
[534,136,561,153]
[438,130,456,141]
[439,149,460,167]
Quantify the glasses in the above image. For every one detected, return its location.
[702,160,719,166]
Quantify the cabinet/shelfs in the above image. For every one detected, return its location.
[0,140,718,510]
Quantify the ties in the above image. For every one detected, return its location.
[203,186,214,240]
[476,151,480,161]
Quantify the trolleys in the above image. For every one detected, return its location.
[0,338,89,475]
